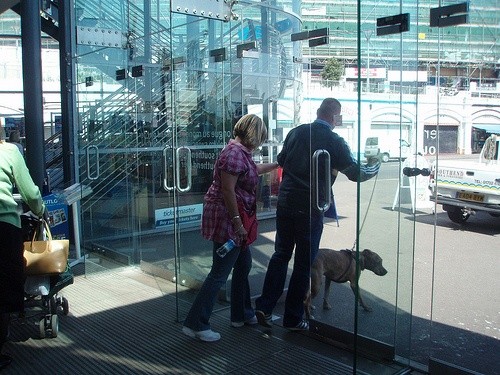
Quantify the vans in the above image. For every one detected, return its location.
[363,136,412,163]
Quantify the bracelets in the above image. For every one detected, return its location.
[276,161,280,168]
[234,224,243,234]
[231,216,241,222]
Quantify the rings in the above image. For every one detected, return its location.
[241,238,243,240]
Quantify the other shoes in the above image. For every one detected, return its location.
[0,354,12,368]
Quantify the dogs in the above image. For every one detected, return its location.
[304,246,390,323]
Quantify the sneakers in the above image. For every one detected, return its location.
[288,319,310,331]
[231,314,258,329]
[256,308,274,328]
[182,325,221,342]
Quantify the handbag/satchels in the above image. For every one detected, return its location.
[22,219,70,277]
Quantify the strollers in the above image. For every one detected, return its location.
[19,210,74,339]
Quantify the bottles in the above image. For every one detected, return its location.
[216,236,240,258]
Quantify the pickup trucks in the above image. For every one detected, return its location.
[429,135,500,224]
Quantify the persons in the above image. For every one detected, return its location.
[0,125,48,347]
[256,98,383,332]
[182,114,283,342]
